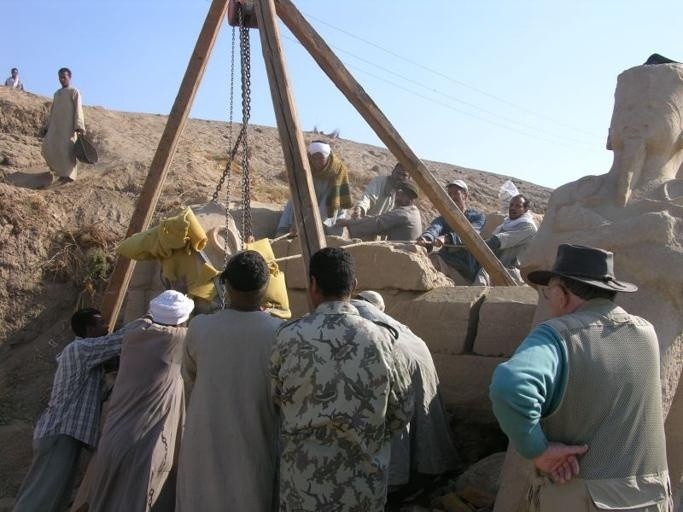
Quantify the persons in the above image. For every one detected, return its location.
[5,68,23,90]
[40,67,84,183]
[9,307,150,511]
[472,194,538,286]
[68,288,194,511]
[275,140,354,239]
[352,163,411,241]
[334,181,422,240]
[488,241,675,512]
[415,180,486,286]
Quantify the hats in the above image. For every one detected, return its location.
[541,282,567,299]
[445,179,469,192]
[526,243,639,294]
